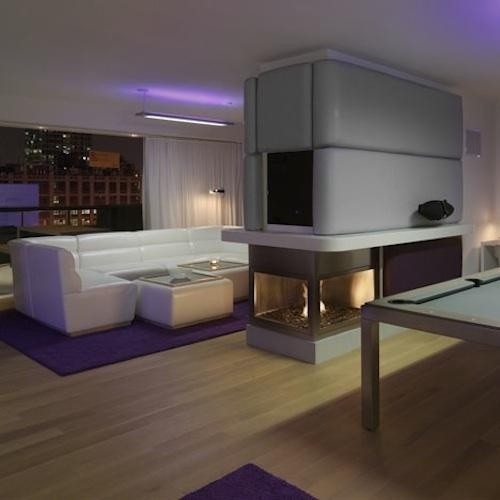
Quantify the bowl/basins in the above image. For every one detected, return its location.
[169,279,190,284]
[167,267,191,280]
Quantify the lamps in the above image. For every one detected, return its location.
[208,185,226,228]
[134,87,234,127]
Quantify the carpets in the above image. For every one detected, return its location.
[178,459,319,499]
[0,299,254,377]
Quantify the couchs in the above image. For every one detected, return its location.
[7,220,253,339]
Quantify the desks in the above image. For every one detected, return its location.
[349,262,499,436]
[478,239,500,273]
